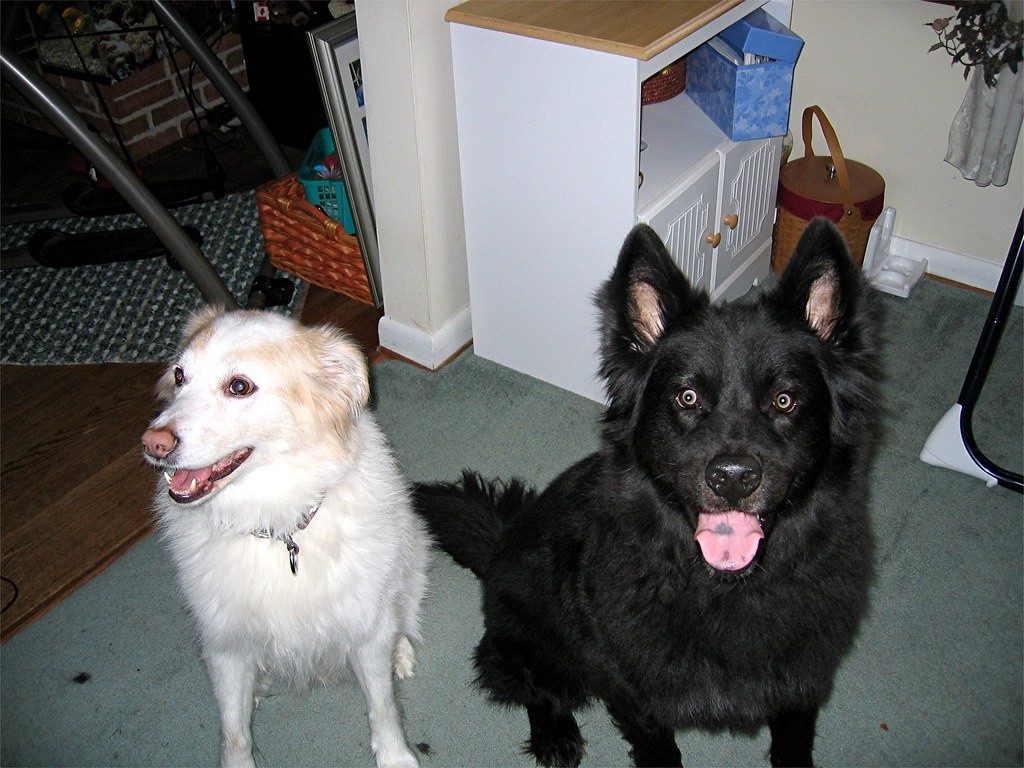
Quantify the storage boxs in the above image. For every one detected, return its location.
[684,8,804,142]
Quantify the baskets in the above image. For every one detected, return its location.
[256,171,377,307]
[295,128,356,236]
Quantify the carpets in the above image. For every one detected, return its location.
[1,191,309,368]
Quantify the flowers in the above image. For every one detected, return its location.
[924,0,1024,90]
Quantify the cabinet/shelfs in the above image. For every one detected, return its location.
[444,1,794,407]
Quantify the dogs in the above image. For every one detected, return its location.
[410,217,908,768]
[140,304,439,768]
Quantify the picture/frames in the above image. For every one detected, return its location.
[307,9,385,311]
